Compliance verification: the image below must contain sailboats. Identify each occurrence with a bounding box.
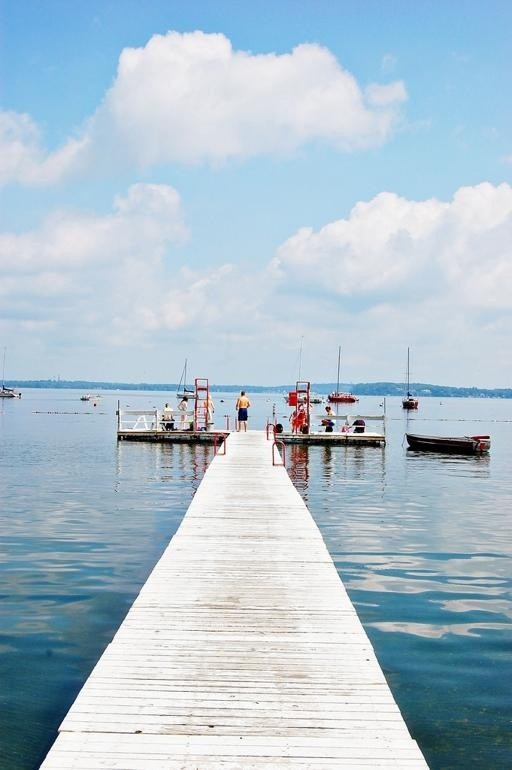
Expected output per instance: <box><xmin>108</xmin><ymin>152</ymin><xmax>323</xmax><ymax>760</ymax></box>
<box><xmin>402</xmin><ymin>347</ymin><xmax>419</xmax><ymax>408</ymax></box>
<box><xmin>176</xmin><ymin>357</ymin><xmax>198</xmax><ymax>398</ymax></box>
<box><xmin>284</xmin><ymin>335</ymin><xmax>359</xmax><ymax>402</ymax></box>
<box><xmin>0</xmin><ymin>347</ymin><xmax>22</xmax><ymax>399</ymax></box>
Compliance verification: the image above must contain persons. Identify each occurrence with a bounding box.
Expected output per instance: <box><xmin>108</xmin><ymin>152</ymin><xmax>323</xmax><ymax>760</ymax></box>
<box><xmin>289</xmin><ymin>397</ymin><xmax>312</xmax><ymax>434</ymax></box>
<box><xmin>320</xmin><ymin>406</ymin><xmax>336</xmax><ymax>432</ymax></box>
<box><xmin>236</xmin><ymin>391</ymin><xmax>251</xmax><ymax>432</ymax></box>
<box><xmin>202</xmin><ymin>395</ymin><xmax>215</xmax><ymax>432</ymax></box>
<box><xmin>161</xmin><ymin>403</ymin><xmax>175</xmax><ymax>431</ymax></box>
<box><xmin>341</xmin><ymin>423</ymin><xmax>351</xmax><ymax>433</ymax></box>
<box><xmin>350</xmin><ymin>415</ymin><xmax>365</xmax><ymax>433</ymax></box>
<box><xmin>177</xmin><ymin>396</ymin><xmax>188</xmax><ymax>430</ymax></box>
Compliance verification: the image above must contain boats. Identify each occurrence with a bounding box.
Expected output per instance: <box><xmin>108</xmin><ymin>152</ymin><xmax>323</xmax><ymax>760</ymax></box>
<box><xmin>405</xmin><ymin>432</ymin><xmax>491</xmax><ymax>454</ymax></box>
<box><xmin>80</xmin><ymin>393</ymin><xmax>105</xmax><ymax>401</ymax></box>
